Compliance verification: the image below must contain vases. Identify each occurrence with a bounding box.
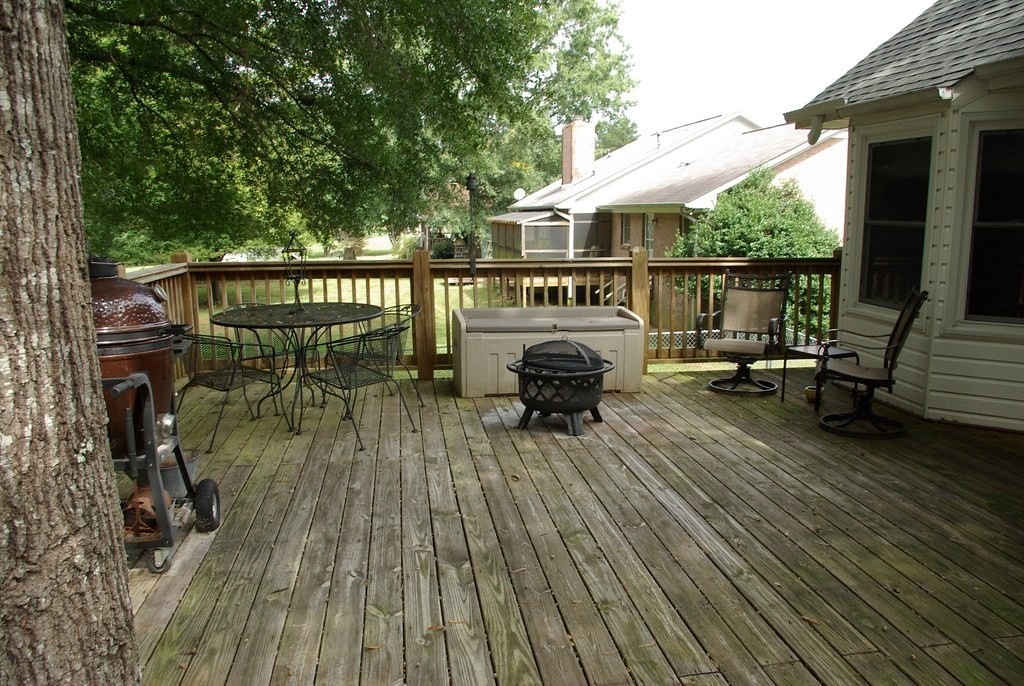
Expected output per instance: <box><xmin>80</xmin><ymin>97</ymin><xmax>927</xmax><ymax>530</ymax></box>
<box><xmin>135</xmin><ymin>450</ymin><xmax>200</xmax><ymax>496</ymax></box>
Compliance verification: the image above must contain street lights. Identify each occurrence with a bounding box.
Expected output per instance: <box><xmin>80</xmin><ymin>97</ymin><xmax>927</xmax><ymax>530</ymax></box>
<box><xmin>463</xmin><ymin>173</ymin><xmax>478</xmax><ymax>257</ymax></box>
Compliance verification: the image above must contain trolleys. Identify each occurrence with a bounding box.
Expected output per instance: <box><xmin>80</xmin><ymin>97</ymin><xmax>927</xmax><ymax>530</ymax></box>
<box><xmin>93</xmin><ymin>371</ymin><xmax>221</xmax><ymax>576</ymax></box>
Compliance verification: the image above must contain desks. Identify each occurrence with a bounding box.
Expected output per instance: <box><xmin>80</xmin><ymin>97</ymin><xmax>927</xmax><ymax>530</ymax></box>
<box><xmin>210</xmin><ymin>302</ymin><xmax>384</xmax><ymax>437</ymax></box>
<box><xmin>782</xmin><ymin>344</ymin><xmax>859</xmax><ymax>408</ymax></box>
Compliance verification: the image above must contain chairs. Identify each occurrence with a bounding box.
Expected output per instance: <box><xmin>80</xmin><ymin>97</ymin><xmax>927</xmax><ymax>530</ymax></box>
<box><xmin>176</xmin><ymin>335</ymin><xmax>291</xmax><ymax>453</ymax></box>
<box><xmin>814</xmin><ymin>283</ymin><xmax>928</xmax><ymax>440</ymax></box>
<box><xmin>223</xmin><ymin>302</ymin><xmax>426</xmax><ymax>451</ymax></box>
<box><xmin>694</xmin><ymin>269</ymin><xmax>792</xmax><ymax>396</ymax></box>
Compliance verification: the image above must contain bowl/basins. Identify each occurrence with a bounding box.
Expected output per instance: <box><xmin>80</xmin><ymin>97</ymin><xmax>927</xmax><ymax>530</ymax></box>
<box><xmin>141</xmin><ymin>448</ymin><xmax>200</xmax><ymax>499</ymax></box>
<box><xmin>806</xmin><ymin>386</ymin><xmax>825</xmax><ymax>403</ymax></box>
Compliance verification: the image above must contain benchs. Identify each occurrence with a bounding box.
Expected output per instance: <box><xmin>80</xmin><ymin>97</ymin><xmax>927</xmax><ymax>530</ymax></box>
<box><xmin>450</xmin><ymin>305</ymin><xmax>645</xmax><ymax>399</ymax></box>
